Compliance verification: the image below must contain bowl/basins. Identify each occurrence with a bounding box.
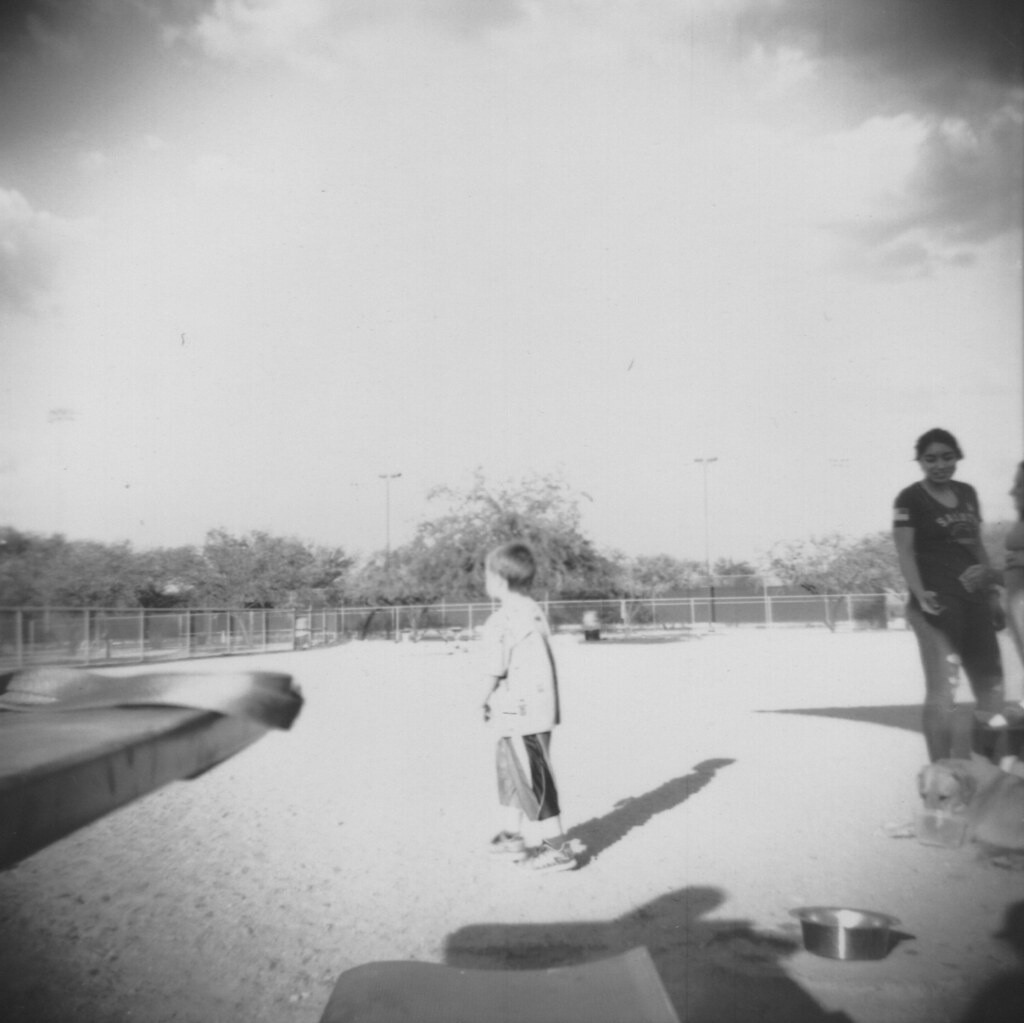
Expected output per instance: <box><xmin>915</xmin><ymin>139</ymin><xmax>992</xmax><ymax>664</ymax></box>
<box><xmin>789</xmin><ymin>907</ymin><xmax>903</xmax><ymax>960</ymax></box>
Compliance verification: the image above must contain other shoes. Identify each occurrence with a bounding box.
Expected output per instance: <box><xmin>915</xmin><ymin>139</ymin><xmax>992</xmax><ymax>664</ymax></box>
<box><xmin>525</xmin><ymin>838</ymin><xmax>578</xmax><ymax>874</ymax></box>
<box><xmin>489</xmin><ymin>831</ymin><xmax>527</xmax><ymax>861</ymax></box>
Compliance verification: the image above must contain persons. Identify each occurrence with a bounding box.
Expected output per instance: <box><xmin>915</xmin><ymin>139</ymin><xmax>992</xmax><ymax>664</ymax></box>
<box><xmin>475</xmin><ymin>544</ymin><xmax>578</xmax><ymax>876</ymax></box>
<box><xmin>1005</xmin><ymin>458</ymin><xmax>1024</xmax><ymax>658</ymax></box>
<box><xmin>894</xmin><ymin>429</ymin><xmax>1024</xmax><ymax>779</ymax></box>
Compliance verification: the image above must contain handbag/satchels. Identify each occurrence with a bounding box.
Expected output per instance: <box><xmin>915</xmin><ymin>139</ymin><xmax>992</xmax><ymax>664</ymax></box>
<box><xmin>944</xmin><ymin>587</ymin><xmax>1007</xmax><ymax>643</ymax></box>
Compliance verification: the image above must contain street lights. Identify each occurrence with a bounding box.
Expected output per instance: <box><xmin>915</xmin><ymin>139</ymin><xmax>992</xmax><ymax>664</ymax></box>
<box><xmin>377</xmin><ymin>469</ymin><xmax>402</xmax><ymax>563</ymax></box>
<box><xmin>692</xmin><ymin>453</ymin><xmax>720</xmax><ymax>582</ymax></box>
